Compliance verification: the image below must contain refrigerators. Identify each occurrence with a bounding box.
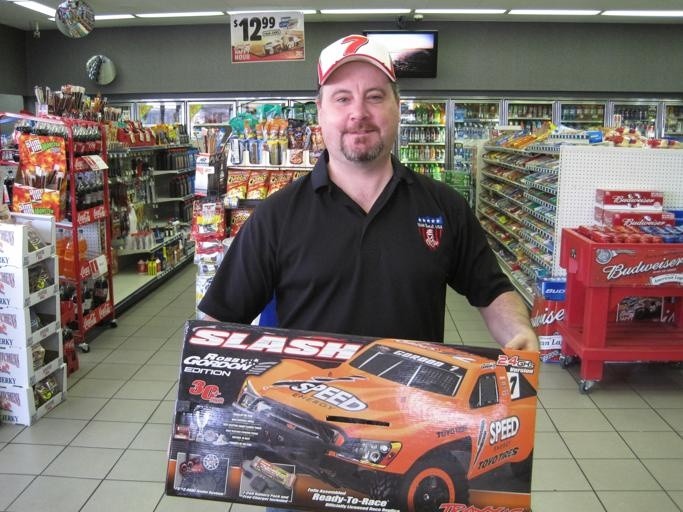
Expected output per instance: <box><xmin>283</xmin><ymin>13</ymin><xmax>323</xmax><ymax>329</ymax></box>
<box><xmin>113</xmin><ymin>98</ymin><xmax>683</xmax><ymax>183</ymax></box>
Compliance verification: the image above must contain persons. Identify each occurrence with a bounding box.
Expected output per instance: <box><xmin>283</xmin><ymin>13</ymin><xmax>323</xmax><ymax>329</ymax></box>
<box><xmin>197</xmin><ymin>34</ymin><xmax>540</xmax><ymax>353</ymax></box>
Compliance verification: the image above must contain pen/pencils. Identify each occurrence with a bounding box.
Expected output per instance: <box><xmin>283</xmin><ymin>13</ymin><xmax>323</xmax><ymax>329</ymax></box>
<box><xmin>35</xmin><ymin>85</ymin><xmax>82</xmax><ymax>116</ymax></box>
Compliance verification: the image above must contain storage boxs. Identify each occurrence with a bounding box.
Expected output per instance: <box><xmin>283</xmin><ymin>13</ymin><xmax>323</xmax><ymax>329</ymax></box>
<box><xmin>166</xmin><ymin>320</ymin><xmax>539</xmax><ymax>512</ymax></box>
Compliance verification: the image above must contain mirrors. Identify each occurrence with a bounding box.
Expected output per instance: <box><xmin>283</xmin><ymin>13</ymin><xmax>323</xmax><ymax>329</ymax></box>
<box><xmin>86</xmin><ymin>55</ymin><xmax>117</xmax><ymax>86</ymax></box>
<box><xmin>55</xmin><ymin>0</ymin><xmax>95</xmax><ymax>39</ymax></box>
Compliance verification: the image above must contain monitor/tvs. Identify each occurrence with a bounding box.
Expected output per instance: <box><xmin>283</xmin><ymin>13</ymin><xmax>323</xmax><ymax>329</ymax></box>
<box><xmin>362</xmin><ymin>28</ymin><xmax>439</xmax><ymax>79</ymax></box>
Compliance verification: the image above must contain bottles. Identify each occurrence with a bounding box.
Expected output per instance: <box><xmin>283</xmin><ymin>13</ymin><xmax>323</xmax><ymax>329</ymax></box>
<box><xmin>136</xmin><ymin>124</ymin><xmax>197</xmax><ymax>276</ymax></box>
<box><xmin>2</xmin><ymin>111</ymin><xmax>112</xmax><ymax>376</ymax></box>
<box><xmin>399</xmin><ymin>105</ymin><xmax>651</xmax><ymax>179</ymax></box>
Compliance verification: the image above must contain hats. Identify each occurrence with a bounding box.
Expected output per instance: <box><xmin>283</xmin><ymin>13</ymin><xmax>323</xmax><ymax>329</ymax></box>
<box><xmin>317</xmin><ymin>34</ymin><xmax>397</xmax><ymax>86</ymax></box>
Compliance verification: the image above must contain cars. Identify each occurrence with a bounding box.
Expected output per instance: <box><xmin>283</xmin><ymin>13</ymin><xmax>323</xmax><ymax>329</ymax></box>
<box><xmin>231</xmin><ymin>340</ymin><xmax>536</xmax><ymax>512</ymax></box>
